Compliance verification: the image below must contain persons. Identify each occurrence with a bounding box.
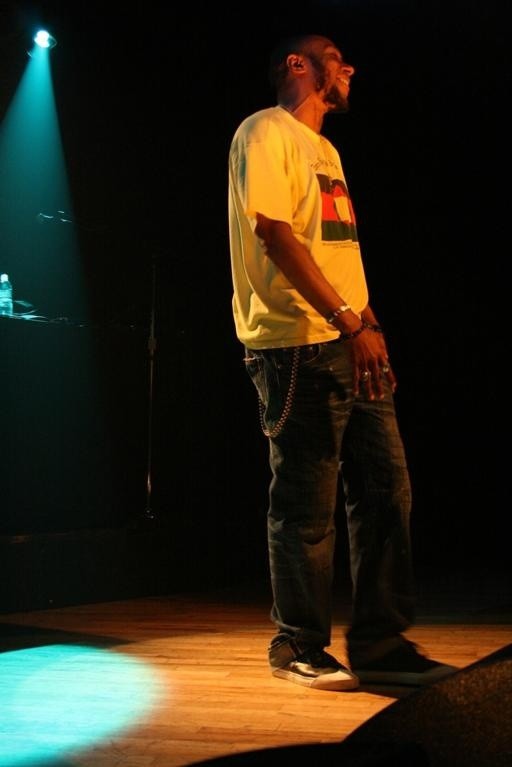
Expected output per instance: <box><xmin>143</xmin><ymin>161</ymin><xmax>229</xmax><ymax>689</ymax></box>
<box><xmin>226</xmin><ymin>33</ymin><xmax>461</xmax><ymax>691</ymax></box>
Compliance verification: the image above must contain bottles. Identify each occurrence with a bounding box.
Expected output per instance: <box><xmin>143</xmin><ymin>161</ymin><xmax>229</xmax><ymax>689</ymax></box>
<box><xmin>0</xmin><ymin>273</ymin><xmax>14</xmax><ymax>317</ymax></box>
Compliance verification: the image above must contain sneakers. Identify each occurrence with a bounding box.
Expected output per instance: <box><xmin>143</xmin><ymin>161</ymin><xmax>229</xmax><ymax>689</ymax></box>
<box><xmin>270</xmin><ymin>647</ymin><xmax>361</xmax><ymax>692</ymax></box>
<box><xmin>348</xmin><ymin>643</ymin><xmax>459</xmax><ymax>688</ymax></box>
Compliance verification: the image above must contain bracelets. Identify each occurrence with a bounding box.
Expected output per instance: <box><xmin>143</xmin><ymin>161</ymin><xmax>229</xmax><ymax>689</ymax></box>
<box><xmin>337</xmin><ymin>321</ymin><xmax>368</xmax><ymax>341</ymax></box>
<box><xmin>326</xmin><ymin>304</ymin><xmax>350</xmax><ymax>323</ymax></box>
<box><xmin>368</xmin><ymin>323</ymin><xmax>382</xmax><ymax>333</ymax></box>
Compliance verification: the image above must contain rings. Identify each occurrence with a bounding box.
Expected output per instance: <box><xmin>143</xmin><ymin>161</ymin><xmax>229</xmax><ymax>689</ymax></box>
<box><xmin>360</xmin><ymin>370</ymin><xmax>373</xmax><ymax>383</ymax></box>
<box><xmin>380</xmin><ymin>361</ymin><xmax>391</xmax><ymax>377</ymax></box>
